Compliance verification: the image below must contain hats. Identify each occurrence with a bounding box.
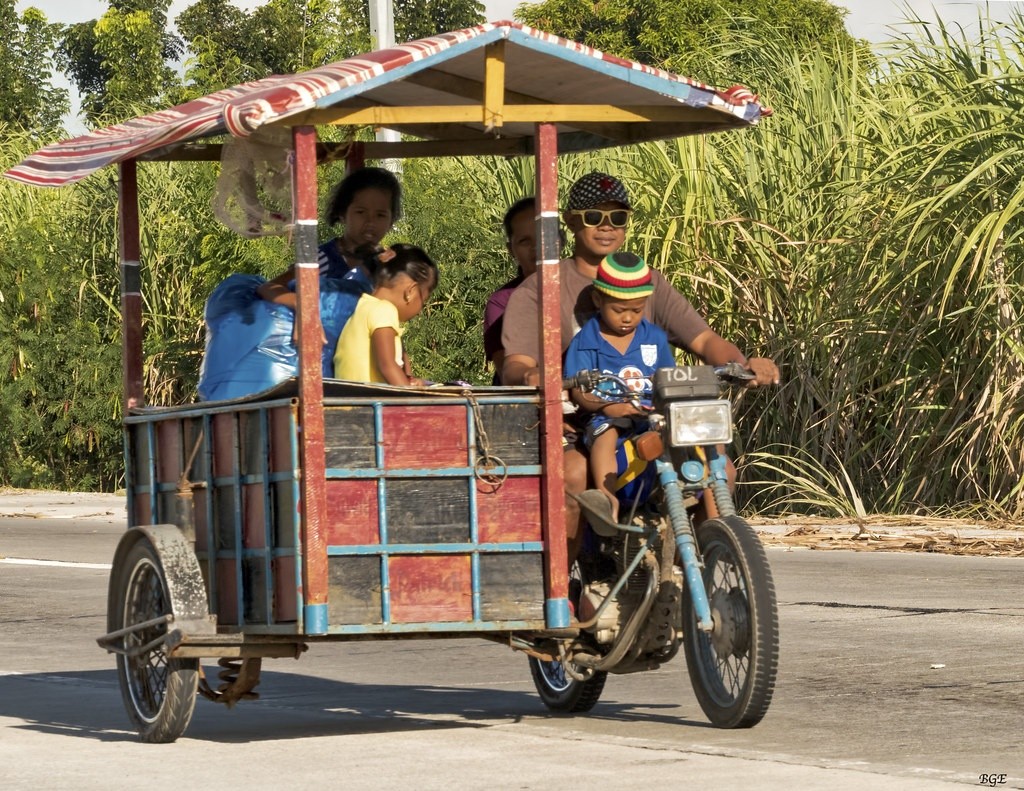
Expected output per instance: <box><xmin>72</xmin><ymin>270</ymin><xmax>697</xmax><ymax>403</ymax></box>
<box><xmin>592</xmin><ymin>252</ymin><xmax>654</xmax><ymax>300</ymax></box>
<box><xmin>567</xmin><ymin>172</ymin><xmax>632</xmax><ymax>210</ymax></box>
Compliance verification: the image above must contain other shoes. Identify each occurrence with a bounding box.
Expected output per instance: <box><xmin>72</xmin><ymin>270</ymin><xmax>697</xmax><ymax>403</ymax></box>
<box><xmin>580</xmin><ymin>489</ymin><xmax>621</xmax><ymax>536</ymax></box>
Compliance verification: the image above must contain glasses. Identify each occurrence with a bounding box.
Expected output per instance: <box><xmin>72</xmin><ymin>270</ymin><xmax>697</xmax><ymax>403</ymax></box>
<box><xmin>570</xmin><ymin>209</ymin><xmax>631</xmax><ymax>227</ymax></box>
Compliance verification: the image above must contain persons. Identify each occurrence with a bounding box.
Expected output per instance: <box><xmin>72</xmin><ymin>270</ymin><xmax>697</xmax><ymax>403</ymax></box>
<box><xmin>482</xmin><ymin>198</ymin><xmax>563</xmax><ymax>387</ymax></box>
<box><xmin>257</xmin><ymin>167</ymin><xmax>402</xmax><ymax>350</ymax></box>
<box><xmin>564</xmin><ymin>251</ymin><xmax>675</xmax><ymax>537</ymax></box>
<box><xmin>332</xmin><ymin>243</ymin><xmax>439</xmax><ymax>389</ymax></box>
<box><xmin>500</xmin><ymin>168</ymin><xmax>780</xmax><ymax>627</ymax></box>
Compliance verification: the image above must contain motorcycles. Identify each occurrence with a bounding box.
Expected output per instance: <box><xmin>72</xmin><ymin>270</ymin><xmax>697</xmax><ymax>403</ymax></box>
<box><xmin>4</xmin><ymin>18</ymin><xmax>781</xmax><ymax>749</ymax></box>
